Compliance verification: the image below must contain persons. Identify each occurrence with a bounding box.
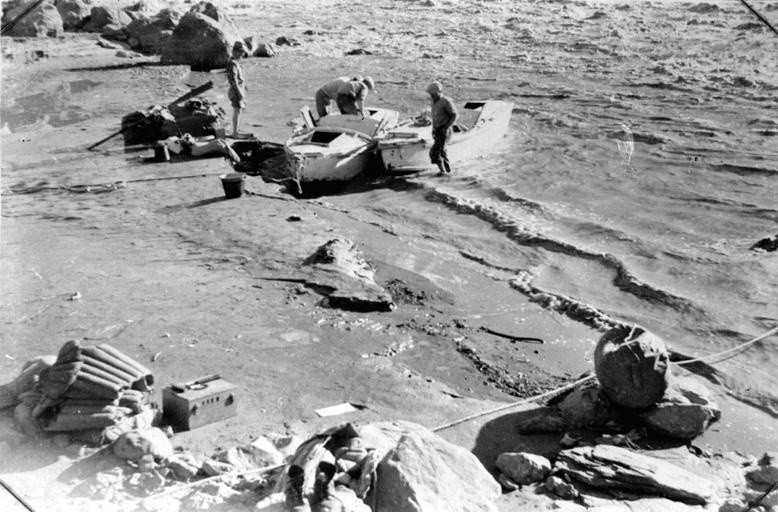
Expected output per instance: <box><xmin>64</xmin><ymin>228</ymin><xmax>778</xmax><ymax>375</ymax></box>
<box><xmin>336</xmin><ymin>75</ymin><xmax>375</xmax><ymax>117</ymax></box>
<box><xmin>226</xmin><ymin>41</ymin><xmax>249</xmax><ymax>140</ymax></box>
<box><xmin>316</xmin><ymin>73</ymin><xmax>361</xmax><ymax>117</ymax></box>
<box><xmin>425</xmin><ymin>81</ymin><xmax>458</xmax><ymax>176</ymax></box>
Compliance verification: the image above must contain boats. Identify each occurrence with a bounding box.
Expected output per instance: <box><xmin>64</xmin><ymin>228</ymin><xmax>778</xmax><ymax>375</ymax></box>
<box><xmin>122</xmin><ymin>104</ymin><xmax>217</xmax><ymax>146</ymax></box>
<box><xmin>284</xmin><ymin>105</ymin><xmax>399</xmax><ymax>182</ymax></box>
<box><xmin>377</xmin><ymin>100</ymin><xmax>512</xmax><ymax>170</ymax></box>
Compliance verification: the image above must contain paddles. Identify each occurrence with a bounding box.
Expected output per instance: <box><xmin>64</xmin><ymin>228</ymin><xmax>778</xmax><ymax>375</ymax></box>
<box><xmin>87</xmin><ymin>80</ymin><xmax>213</xmax><ymax>150</ymax></box>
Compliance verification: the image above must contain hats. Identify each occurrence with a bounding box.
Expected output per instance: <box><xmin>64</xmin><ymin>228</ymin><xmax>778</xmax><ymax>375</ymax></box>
<box><xmin>426</xmin><ymin>81</ymin><xmax>442</xmax><ymax>94</ymax></box>
<box><xmin>353</xmin><ymin>75</ymin><xmax>374</xmax><ymax>90</ymax></box>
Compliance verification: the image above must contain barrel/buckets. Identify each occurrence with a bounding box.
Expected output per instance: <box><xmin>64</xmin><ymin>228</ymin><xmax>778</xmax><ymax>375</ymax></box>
<box><xmin>155</xmin><ymin>143</ymin><xmax>170</xmax><ymax>162</ymax></box>
<box><xmin>220</xmin><ymin>173</ymin><xmax>246</xmax><ymax>198</ymax></box>
